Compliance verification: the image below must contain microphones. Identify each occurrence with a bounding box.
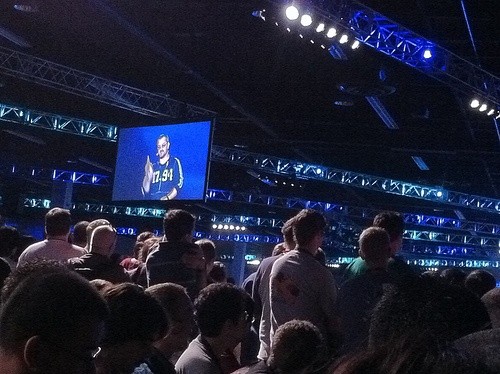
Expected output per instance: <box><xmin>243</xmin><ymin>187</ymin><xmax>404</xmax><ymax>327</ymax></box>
<box><xmin>156</xmin><ymin>153</ymin><xmax>159</xmax><ymax>156</ymax></box>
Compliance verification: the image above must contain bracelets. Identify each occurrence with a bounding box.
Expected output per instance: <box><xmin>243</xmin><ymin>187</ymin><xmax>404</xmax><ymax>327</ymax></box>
<box><xmin>166</xmin><ymin>195</ymin><xmax>169</xmax><ymax>200</ymax></box>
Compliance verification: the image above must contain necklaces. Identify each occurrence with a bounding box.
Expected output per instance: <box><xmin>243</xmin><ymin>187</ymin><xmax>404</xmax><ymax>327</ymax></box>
<box><xmin>48</xmin><ymin>238</ymin><xmax>67</xmax><ymax>242</ymax></box>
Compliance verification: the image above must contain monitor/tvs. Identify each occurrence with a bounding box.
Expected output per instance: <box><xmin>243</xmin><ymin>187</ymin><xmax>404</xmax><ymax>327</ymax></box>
<box><xmin>112</xmin><ymin>117</ymin><xmax>215</xmax><ymax>205</ymax></box>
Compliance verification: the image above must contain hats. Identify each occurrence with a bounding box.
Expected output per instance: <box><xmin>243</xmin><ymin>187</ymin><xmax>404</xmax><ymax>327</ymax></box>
<box><xmin>281</xmin><ymin>216</ymin><xmax>296</xmax><ymax>234</ymax></box>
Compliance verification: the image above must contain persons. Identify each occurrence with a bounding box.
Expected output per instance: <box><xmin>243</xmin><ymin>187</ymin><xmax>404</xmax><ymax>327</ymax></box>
<box><xmin>140</xmin><ymin>132</ymin><xmax>185</xmax><ymax>200</ymax></box>
<box><xmin>0</xmin><ymin>207</ymin><xmax>500</xmax><ymax>374</ymax></box>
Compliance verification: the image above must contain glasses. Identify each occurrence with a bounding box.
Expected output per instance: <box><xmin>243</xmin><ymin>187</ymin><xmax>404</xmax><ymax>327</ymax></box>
<box><xmin>156</xmin><ymin>142</ymin><xmax>167</xmax><ymax>150</ymax></box>
<box><xmin>27</xmin><ymin>331</ymin><xmax>102</xmax><ymax>364</ymax></box>
<box><xmin>224</xmin><ymin>314</ymin><xmax>255</xmax><ymax>326</ymax></box>
